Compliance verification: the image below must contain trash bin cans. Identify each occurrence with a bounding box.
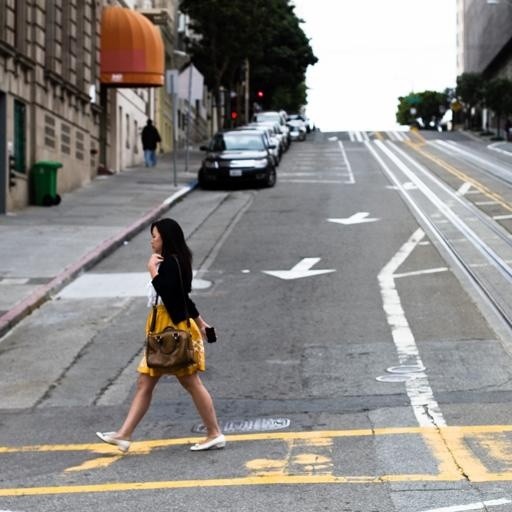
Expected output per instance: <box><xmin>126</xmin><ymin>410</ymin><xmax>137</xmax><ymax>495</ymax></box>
<box><xmin>30</xmin><ymin>161</ymin><xmax>61</xmax><ymax>206</ymax></box>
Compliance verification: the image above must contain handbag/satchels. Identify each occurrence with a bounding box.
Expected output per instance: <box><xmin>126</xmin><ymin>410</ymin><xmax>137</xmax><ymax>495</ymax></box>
<box><xmin>146</xmin><ymin>326</ymin><xmax>196</xmax><ymax>368</ymax></box>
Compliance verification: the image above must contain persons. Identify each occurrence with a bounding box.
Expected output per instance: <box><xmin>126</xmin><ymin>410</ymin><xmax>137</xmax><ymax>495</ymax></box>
<box><xmin>95</xmin><ymin>217</ymin><xmax>226</xmax><ymax>453</ymax></box>
<box><xmin>139</xmin><ymin>117</ymin><xmax>165</xmax><ymax>168</ymax></box>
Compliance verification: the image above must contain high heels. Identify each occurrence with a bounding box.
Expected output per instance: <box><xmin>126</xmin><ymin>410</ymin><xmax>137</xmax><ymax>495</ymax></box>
<box><xmin>190</xmin><ymin>433</ymin><xmax>226</xmax><ymax>451</ymax></box>
<box><xmin>96</xmin><ymin>431</ymin><xmax>132</xmax><ymax>453</ymax></box>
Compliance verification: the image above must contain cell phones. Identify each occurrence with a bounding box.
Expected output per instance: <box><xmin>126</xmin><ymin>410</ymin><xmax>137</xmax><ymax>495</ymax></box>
<box><xmin>205</xmin><ymin>327</ymin><xmax>216</xmax><ymax>343</ymax></box>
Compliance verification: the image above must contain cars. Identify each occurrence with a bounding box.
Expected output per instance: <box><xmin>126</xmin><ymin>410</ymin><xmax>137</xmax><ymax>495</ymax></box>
<box><xmin>232</xmin><ymin>106</ymin><xmax>310</xmax><ymax>164</ymax></box>
<box><xmin>196</xmin><ymin>126</ymin><xmax>279</xmax><ymax>190</ymax></box>
<box><xmin>409</xmin><ymin>112</ymin><xmax>449</xmax><ymax>133</ymax></box>
